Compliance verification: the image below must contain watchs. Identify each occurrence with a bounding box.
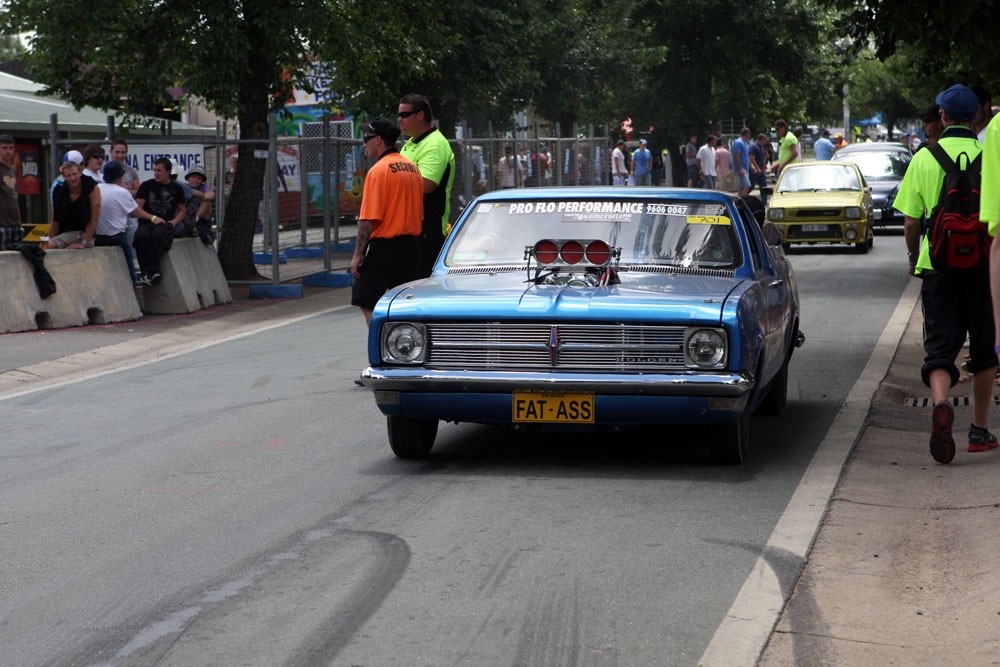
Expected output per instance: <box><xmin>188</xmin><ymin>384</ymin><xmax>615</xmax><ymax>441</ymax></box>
<box><xmin>907</xmin><ymin>250</ymin><xmax>920</xmax><ymax>265</ymax></box>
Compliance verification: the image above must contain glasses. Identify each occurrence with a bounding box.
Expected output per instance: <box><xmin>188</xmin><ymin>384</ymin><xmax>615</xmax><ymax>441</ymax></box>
<box><xmin>363</xmin><ymin>134</ymin><xmax>377</xmax><ymax>143</ymax></box>
<box><xmin>86</xmin><ymin>153</ymin><xmax>105</xmax><ymax>160</ymax></box>
<box><xmin>923</xmin><ymin>115</ymin><xmax>939</xmax><ymax>123</ymax></box>
<box><xmin>398</xmin><ymin>109</ymin><xmax>422</xmax><ymax>118</ymax></box>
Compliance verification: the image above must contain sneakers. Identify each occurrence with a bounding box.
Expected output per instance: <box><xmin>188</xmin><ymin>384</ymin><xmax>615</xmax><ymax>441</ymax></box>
<box><xmin>929</xmin><ymin>400</ymin><xmax>956</xmax><ymax>464</ymax></box>
<box><xmin>968</xmin><ymin>423</ymin><xmax>998</xmax><ymax>452</ymax></box>
<box><xmin>151</xmin><ymin>270</ymin><xmax>162</xmax><ymax>286</ymax></box>
<box><xmin>140</xmin><ymin>271</ymin><xmax>152</xmax><ymax>288</ymax></box>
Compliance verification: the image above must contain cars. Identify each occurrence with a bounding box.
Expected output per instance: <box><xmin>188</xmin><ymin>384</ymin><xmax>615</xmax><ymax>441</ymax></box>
<box><xmin>363</xmin><ymin>181</ymin><xmax>801</xmax><ymax>467</ymax></box>
<box><xmin>831</xmin><ymin>141</ymin><xmax>915</xmax><ymax>233</ymax></box>
<box><xmin>759</xmin><ymin>160</ymin><xmax>876</xmax><ymax>254</ymax></box>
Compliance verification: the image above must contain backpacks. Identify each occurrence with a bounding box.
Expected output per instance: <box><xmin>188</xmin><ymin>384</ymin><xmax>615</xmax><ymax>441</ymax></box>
<box><xmin>923</xmin><ymin>142</ymin><xmax>993</xmax><ymax>273</ymax></box>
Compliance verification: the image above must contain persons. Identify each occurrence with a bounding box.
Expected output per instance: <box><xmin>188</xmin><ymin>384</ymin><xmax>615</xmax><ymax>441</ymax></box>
<box><xmin>978</xmin><ymin>110</ymin><xmax>1000</xmax><ymax>365</ymax></box>
<box><xmin>970</xmin><ymin>84</ymin><xmax>995</xmax><ymax>144</ymax></box>
<box><xmin>396</xmin><ymin>92</ymin><xmax>457</xmax><ymax>270</ymax></box>
<box><xmin>917</xmin><ymin>104</ymin><xmax>946</xmax><ymax>153</ymax></box>
<box><xmin>0</xmin><ymin>132</ymin><xmax>216</xmax><ymax>287</ymax></box>
<box><xmin>349</xmin><ymin>116</ymin><xmax>426</xmax><ymax>388</ymax></box>
<box><xmin>892</xmin><ymin>84</ymin><xmax>1000</xmax><ymax>464</ymax></box>
<box><xmin>498</xmin><ymin>118</ymin><xmax>922</xmax><ymax>197</ymax></box>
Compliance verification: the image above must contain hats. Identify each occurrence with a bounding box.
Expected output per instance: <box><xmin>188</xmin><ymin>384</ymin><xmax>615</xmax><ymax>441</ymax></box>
<box><xmin>103</xmin><ymin>160</ymin><xmax>125</xmax><ymax>182</ymax></box>
<box><xmin>935</xmin><ymin>83</ymin><xmax>980</xmax><ymax>121</ymax></box>
<box><xmin>359</xmin><ymin>117</ymin><xmax>401</xmax><ymax>141</ymax></box>
<box><xmin>59</xmin><ymin>150</ymin><xmax>84</xmax><ymax>173</ymax></box>
<box><xmin>169</xmin><ymin>158</ymin><xmax>184</xmax><ymax>175</ymax></box>
<box><xmin>185</xmin><ymin>165</ymin><xmax>207</xmax><ymax>182</ymax></box>
